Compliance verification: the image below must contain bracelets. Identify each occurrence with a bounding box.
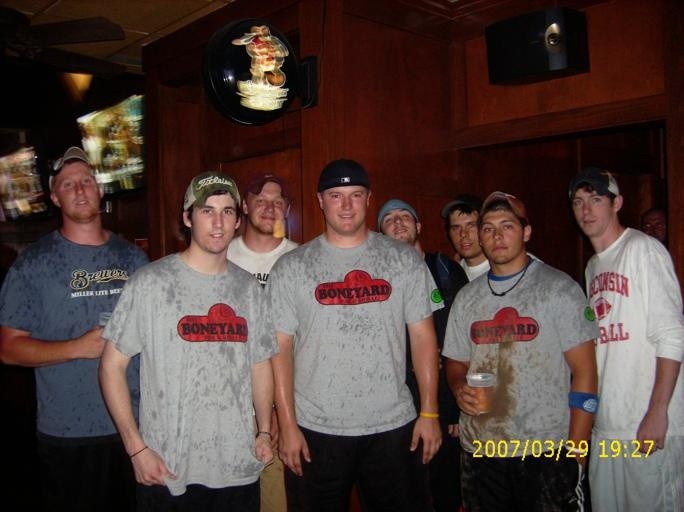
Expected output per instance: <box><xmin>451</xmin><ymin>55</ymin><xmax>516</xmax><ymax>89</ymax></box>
<box><xmin>564</xmin><ymin>389</ymin><xmax>600</xmax><ymax>414</ymax></box>
<box><xmin>252</xmin><ymin>431</ymin><xmax>276</xmax><ymax>439</ymax></box>
<box><xmin>417</xmin><ymin>412</ymin><xmax>445</xmax><ymax>420</ymax></box>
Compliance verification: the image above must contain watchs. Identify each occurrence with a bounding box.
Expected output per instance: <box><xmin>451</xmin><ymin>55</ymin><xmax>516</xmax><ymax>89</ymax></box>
<box><xmin>130</xmin><ymin>444</ymin><xmax>153</xmax><ymax>461</ymax></box>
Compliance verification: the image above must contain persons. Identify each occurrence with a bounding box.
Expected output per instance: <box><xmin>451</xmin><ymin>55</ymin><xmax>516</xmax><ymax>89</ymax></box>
<box><xmin>261</xmin><ymin>159</ymin><xmax>447</xmax><ymax>512</ymax></box>
<box><xmin>377</xmin><ymin>196</ymin><xmax>469</xmax><ymax>512</ymax></box>
<box><xmin>0</xmin><ymin>146</ymin><xmax>155</xmax><ymax>512</ymax></box>
<box><xmin>439</xmin><ymin>192</ymin><xmax>603</xmax><ymax>511</ymax></box>
<box><xmin>224</xmin><ymin>171</ymin><xmax>301</xmax><ymax>512</ymax></box>
<box><xmin>564</xmin><ymin>156</ymin><xmax>683</xmax><ymax>512</ymax></box>
<box><xmin>641</xmin><ymin>204</ymin><xmax>669</xmax><ymax>250</ymax></box>
<box><xmin>98</xmin><ymin>171</ymin><xmax>282</xmax><ymax>512</ymax></box>
<box><xmin>439</xmin><ymin>192</ymin><xmax>542</xmax><ymax>294</ymax></box>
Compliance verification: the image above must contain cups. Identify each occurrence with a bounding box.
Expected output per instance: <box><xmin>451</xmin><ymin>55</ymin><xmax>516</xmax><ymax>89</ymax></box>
<box><xmin>464</xmin><ymin>371</ymin><xmax>498</xmax><ymax>415</ymax></box>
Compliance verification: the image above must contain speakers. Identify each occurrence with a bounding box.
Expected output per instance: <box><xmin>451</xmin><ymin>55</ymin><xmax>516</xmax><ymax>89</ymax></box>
<box><xmin>484</xmin><ymin>8</ymin><xmax>589</xmax><ymax>85</ymax></box>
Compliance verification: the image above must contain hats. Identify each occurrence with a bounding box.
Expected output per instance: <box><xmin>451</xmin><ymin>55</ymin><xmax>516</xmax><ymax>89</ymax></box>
<box><xmin>377</xmin><ymin>199</ymin><xmax>419</xmax><ymax>234</ymax></box>
<box><xmin>568</xmin><ymin>167</ymin><xmax>619</xmax><ymax>200</ymax></box>
<box><xmin>48</xmin><ymin>146</ymin><xmax>96</xmax><ymax>192</ymax></box>
<box><xmin>479</xmin><ymin>191</ymin><xmax>529</xmax><ymax>224</ymax></box>
<box><xmin>245</xmin><ymin>171</ymin><xmax>288</xmax><ymax>198</ymax></box>
<box><xmin>183</xmin><ymin>170</ymin><xmax>240</xmax><ymax>212</ymax></box>
<box><xmin>318</xmin><ymin>159</ymin><xmax>369</xmax><ymax>193</ymax></box>
<box><xmin>440</xmin><ymin>193</ymin><xmax>480</xmax><ymax>219</ymax></box>
